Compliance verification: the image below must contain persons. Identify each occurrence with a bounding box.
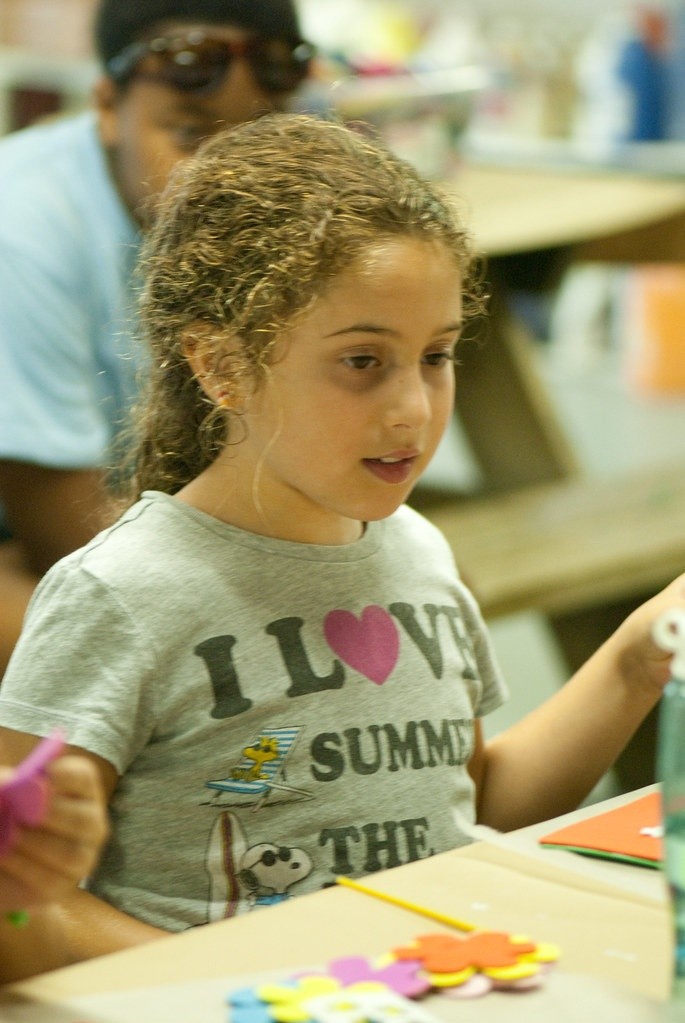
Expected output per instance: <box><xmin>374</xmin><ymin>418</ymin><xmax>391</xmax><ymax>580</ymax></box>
<box><xmin>0</xmin><ymin>115</ymin><xmax>685</xmax><ymax>983</ymax></box>
<box><xmin>0</xmin><ymin>0</ymin><xmax>314</xmax><ymax>682</ymax></box>
<box><xmin>0</xmin><ymin>756</ymin><xmax>105</xmax><ymax>916</ymax></box>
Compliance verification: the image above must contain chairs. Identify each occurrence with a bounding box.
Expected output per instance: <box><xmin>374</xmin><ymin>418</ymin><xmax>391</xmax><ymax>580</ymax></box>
<box><xmin>439</xmin><ymin>462</ymin><xmax>685</xmax><ymax>618</ymax></box>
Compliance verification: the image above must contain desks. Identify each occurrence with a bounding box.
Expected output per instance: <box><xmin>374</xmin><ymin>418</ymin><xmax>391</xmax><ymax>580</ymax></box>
<box><xmin>427</xmin><ymin>173</ymin><xmax>685</xmax><ymax>795</ymax></box>
<box><xmin>0</xmin><ymin>780</ymin><xmax>672</xmax><ymax>1023</ymax></box>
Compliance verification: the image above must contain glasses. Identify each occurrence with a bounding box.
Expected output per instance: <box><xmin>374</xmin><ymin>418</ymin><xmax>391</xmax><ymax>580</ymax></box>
<box><xmin>109</xmin><ymin>27</ymin><xmax>318</xmax><ymax>96</ymax></box>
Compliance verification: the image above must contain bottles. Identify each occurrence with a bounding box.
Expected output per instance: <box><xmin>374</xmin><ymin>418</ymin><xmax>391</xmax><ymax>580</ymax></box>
<box><xmin>654</xmin><ymin>612</ymin><xmax>685</xmax><ymax>997</ymax></box>
